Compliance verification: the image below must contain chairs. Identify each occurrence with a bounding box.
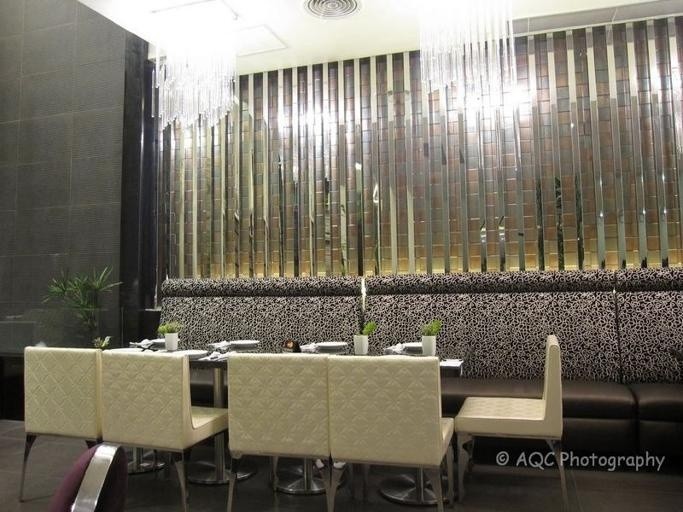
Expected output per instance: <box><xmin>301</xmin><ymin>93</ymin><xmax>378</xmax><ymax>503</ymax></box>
<box><xmin>18</xmin><ymin>346</ymin><xmax>457</xmax><ymax>512</ymax></box>
<box><xmin>453</xmin><ymin>331</ymin><xmax>577</xmax><ymax>511</ymax></box>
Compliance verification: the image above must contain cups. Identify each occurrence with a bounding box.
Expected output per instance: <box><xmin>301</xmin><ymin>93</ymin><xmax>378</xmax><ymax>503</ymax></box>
<box><xmin>422</xmin><ymin>335</ymin><xmax>436</xmax><ymax>356</ymax></box>
<box><xmin>354</xmin><ymin>334</ymin><xmax>368</xmax><ymax>355</ymax></box>
<box><xmin>164</xmin><ymin>333</ymin><xmax>178</xmax><ymax>351</ymax></box>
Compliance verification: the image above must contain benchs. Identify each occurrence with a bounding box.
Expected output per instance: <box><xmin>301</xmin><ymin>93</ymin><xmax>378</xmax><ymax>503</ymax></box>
<box><xmin>154</xmin><ymin>265</ymin><xmax>683</xmax><ymax>464</ymax></box>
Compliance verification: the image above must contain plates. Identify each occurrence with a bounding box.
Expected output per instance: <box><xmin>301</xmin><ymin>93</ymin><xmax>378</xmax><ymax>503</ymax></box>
<box><xmin>403</xmin><ymin>342</ymin><xmax>422</xmax><ymax>351</ymax></box>
<box><xmin>314</xmin><ymin>342</ymin><xmax>347</xmax><ymax>350</ymax></box>
<box><xmin>110</xmin><ymin>339</ymin><xmax>260</xmax><ymax>359</ymax></box>
